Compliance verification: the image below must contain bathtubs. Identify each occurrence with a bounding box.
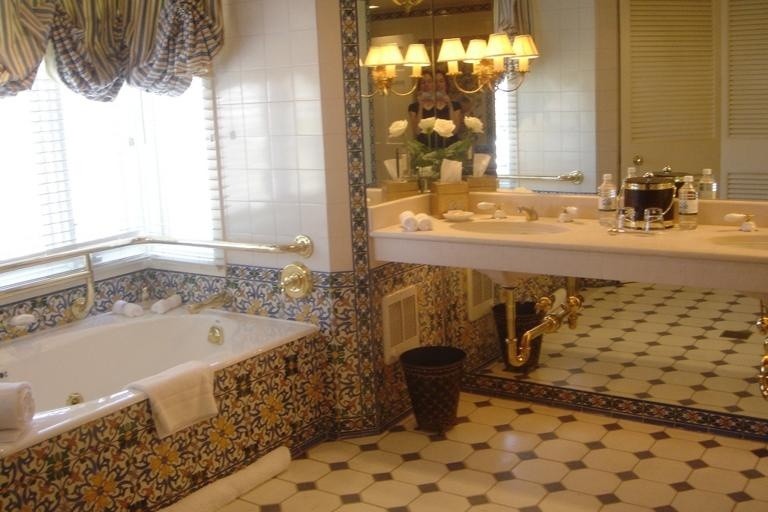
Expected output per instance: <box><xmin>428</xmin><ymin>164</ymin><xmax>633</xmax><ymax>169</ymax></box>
<box><xmin>0</xmin><ymin>299</ymin><xmax>330</xmax><ymax>512</ymax></box>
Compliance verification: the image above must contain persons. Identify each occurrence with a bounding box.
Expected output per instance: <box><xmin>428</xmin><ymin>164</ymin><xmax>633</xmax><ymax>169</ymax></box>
<box><xmin>408</xmin><ymin>70</ymin><xmax>462</xmax><ymax>149</ymax></box>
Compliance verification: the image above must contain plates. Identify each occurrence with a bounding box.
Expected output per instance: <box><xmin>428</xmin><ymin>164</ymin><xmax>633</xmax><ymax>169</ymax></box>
<box><xmin>442</xmin><ymin>211</ymin><xmax>475</xmax><ymax>222</ymax></box>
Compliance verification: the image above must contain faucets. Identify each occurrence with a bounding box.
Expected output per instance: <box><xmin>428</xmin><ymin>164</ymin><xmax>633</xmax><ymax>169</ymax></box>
<box><xmin>185</xmin><ymin>291</ymin><xmax>231</xmax><ymax>315</ymax></box>
<box><xmin>515</xmin><ymin>205</ymin><xmax>539</xmax><ymax>221</ymax></box>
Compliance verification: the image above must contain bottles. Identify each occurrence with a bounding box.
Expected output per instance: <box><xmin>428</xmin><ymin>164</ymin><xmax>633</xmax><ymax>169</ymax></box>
<box><xmin>678</xmin><ymin>175</ymin><xmax>700</xmax><ymax>231</ymax></box>
<box><xmin>627</xmin><ymin>166</ymin><xmax>637</xmax><ymax>179</ymax></box>
<box><xmin>597</xmin><ymin>172</ymin><xmax>617</xmax><ymax>225</ymax></box>
<box><xmin>699</xmin><ymin>168</ymin><xmax>717</xmax><ymax>200</ymax></box>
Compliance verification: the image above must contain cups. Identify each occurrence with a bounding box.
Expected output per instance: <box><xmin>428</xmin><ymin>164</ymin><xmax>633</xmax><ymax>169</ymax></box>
<box><xmin>643</xmin><ymin>207</ymin><xmax>666</xmax><ymax>233</ymax></box>
<box><xmin>616</xmin><ymin>206</ymin><xmax>637</xmax><ymax>233</ymax></box>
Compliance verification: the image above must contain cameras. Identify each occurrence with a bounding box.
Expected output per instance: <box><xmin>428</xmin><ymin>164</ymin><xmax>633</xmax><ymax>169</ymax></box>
<box><xmin>436</xmin><ymin>90</ymin><xmax>445</xmax><ymax>102</ymax></box>
<box><xmin>417</xmin><ymin>90</ymin><xmax>429</xmax><ymax>104</ymax></box>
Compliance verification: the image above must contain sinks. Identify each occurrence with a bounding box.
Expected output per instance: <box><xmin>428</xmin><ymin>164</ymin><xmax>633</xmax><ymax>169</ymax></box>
<box><xmin>711</xmin><ymin>232</ymin><xmax>767</xmax><ymax>250</ymax></box>
<box><xmin>450</xmin><ymin>217</ymin><xmax>572</xmax><ymax>237</ymax></box>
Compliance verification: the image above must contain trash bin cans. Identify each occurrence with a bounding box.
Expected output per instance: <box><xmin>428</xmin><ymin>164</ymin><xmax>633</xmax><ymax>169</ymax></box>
<box><xmin>492</xmin><ymin>301</ymin><xmax>545</xmax><ymax>371</ymax></box>
<box><xmin>400</xmin><ymin>346</ymin><xmax>467</xmax><ymax>432</ymax></box>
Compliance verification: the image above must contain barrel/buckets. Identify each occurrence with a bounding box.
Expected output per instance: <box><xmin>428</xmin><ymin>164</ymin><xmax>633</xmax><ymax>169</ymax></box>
<box><xmin>619</xmin><ymin>176</ymin><xmax>678</xmax><ymax>232</ymax></box>
<box><xmin>653</xmin><ymin>170</ymin><xmax>697</xmax><ymax>199</ymax></box>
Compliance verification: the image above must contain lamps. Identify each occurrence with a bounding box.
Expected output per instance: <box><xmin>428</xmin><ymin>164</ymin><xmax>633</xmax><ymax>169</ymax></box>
<box><xmin>437</xmin><ymin>32</ymin><xmax>539</xmax><ymax>94</ymax></box>
<box><xmin>361</xmin><ymin>44</ymin><xmax>431</xmax><ymax>98</ymax></box>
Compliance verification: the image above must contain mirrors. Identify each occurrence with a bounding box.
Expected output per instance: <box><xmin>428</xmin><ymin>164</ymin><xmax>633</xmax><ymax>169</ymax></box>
<box><xmin>357</xmin><ymin>0</ymin><xmax>768</xmax><ymax>206</ymax></box>
<box><xmin>443</xmin><ymin>266</ymin><xmax>768</xmax><ymax>419</ymax></box>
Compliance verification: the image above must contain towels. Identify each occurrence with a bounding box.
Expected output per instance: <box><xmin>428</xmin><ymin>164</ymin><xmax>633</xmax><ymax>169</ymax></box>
<box><xmin>399</xmin><ymin>211</ymin><xmax>418</xmax><ymax>232</ymax></box>
<box><xmin>125</xmin><ymin>358</ymin><xmax>219</xmax><ymax>440</ymax></box>
<box><xmin>416</xmin><ymin>213</ymin><xmax>433</xmax><ymax>231</ymax></box>
<box><xmin>0</xmin><ymin>382</ymin><xmax>35</xmax><ymax>442</ymax></box>
<box><xmin>112</xmin><ymin>294</ymin><xmax>182</xmax><ymax>317</ymax></box>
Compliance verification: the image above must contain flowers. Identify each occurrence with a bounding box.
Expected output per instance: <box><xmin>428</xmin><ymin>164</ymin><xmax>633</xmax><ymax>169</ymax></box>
<box><xmin>388</xmin><ymin>116</ymin><xmax>484</xmax><ymax>171</ymax></box>
